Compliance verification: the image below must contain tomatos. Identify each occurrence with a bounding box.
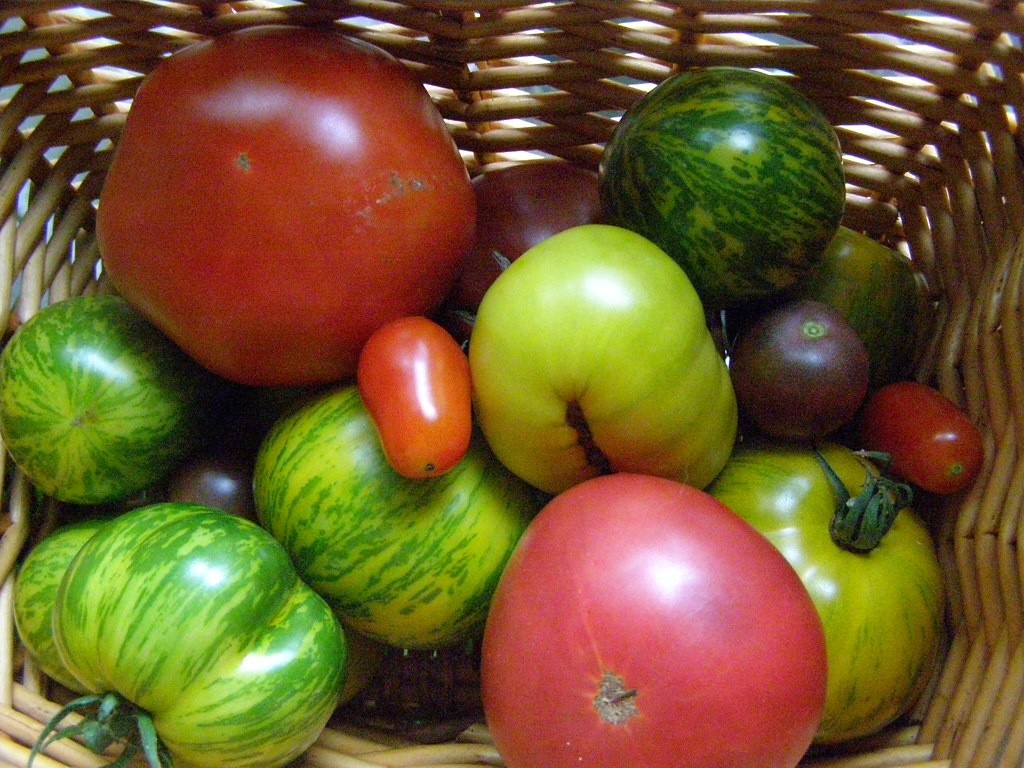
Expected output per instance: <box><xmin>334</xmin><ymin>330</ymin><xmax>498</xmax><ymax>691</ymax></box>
<box><xmin>0</xmin><ymin>21</ymin><xmax>986</xmax><ymax>768</ymax></box>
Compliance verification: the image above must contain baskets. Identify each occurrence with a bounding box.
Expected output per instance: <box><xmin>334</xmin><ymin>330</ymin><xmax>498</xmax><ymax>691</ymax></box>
<box><xmin>0</xmin><ymin>0</ymin><xmax>1024</xmax><ymax>768</ymax></box>
